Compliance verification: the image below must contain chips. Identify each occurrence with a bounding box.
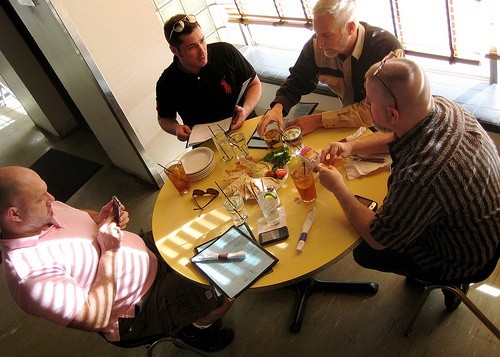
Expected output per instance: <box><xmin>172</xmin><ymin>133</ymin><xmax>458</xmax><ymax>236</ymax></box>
<box><xmin>220</xmin><ymin>156</ymin><xmax>261</xmax><ymax>201</ymax></box>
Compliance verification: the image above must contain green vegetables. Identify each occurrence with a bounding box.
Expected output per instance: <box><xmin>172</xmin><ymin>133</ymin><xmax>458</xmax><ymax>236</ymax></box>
<box><xmin>263</xmin><ymin>146</ymin><xmax>291</xmax><ymax>170</ymax></box>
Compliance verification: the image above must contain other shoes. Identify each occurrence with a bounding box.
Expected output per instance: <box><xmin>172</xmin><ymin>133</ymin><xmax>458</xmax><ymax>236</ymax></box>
<box><xmin>172</xmin><ymin>324</ymin><xmax>235</xmax><ymax>353</ymax></box>
<box><xmin>404</xmin><ymin>276</ymin><xmax>434</xmax><ymax>288</ymax></box>
<box><xmin>442</xmin><ymin>283</ymin><xmax>469</xmax><ymax>312</ymax></box>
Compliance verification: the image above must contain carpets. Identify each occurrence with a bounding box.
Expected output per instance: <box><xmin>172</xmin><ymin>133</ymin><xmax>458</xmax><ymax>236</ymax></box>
<box><xmin>28</xmin><ymin>148</ymin><xmax>104</xmax><ymax>204</ymax></box>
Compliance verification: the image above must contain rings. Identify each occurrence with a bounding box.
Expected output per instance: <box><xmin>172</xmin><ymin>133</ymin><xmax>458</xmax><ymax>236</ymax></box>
<box><xmin>114</xmin><ymin>227</ymin><xmax>121</xmax><ymax>232</ymax></box>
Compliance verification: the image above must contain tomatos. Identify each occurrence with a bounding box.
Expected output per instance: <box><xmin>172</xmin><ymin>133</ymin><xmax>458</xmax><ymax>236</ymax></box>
<box><xmin>275</xmin><ymin>168</ymin><xmax>287</xmax><ymax>177</ymax></box>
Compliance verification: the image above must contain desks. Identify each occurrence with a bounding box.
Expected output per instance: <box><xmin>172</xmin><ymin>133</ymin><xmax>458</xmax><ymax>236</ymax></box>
<box><xmin>151</xmin><ymin>110</ymin><xmax>393</xmax><ymax>334</ymax></box>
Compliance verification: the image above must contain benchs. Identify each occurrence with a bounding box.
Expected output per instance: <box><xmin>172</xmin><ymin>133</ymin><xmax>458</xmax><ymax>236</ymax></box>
<box><xmin>230</xmin><ymin>43</ymin><xmax>500</xmax><ymax>157</ymax></box>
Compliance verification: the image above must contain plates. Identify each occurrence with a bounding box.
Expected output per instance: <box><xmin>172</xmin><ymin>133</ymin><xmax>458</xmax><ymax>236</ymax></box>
<box><xmin>179</xmin><ymin>147</ymin><xmax>216</xmax><ymax>181</ymax></box>
<box><xmin>245</xmin><ymin>173</ymin><xmax>288</xmax><ymax>199</ymax></box>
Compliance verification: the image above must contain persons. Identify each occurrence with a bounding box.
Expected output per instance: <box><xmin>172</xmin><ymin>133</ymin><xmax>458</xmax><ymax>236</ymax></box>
<box><xmin>155</xmin><ymin>14</ymin><xmax>262</xmax><ymax>149</ymax></box>
<box><xmin>0</xmin><ymin>166</ymin><xmax>234</xmax><ymax>353</ymax></box>
<box><xmin>257</xmin><ymin>0</ymin><xmax>405</xmax><ymax>136</ymax></box>
<box><xmin>315</xmin><ymin>51</ymin><xmax>500</xmax><ymax>311</ymax></box>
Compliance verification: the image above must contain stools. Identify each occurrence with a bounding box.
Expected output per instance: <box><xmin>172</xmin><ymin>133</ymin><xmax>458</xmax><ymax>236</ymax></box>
<box><xmin>401</xmin><ymin>244</ymin><xmax>500</xmax><ymax>339</ymax></box>
<box><xmin>125</xmin><ymin>325</ymin><xmax>210</xmax><ymax>357</ymax></box>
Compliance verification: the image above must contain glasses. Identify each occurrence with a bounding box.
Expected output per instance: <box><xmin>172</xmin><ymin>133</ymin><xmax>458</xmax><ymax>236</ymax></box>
<box><xmin>373</xmin><ymin>51</ymin><xmax>398</xmax><ymax>111</ymax></box>
<box><xmin>169</xmin><ymin>13</ymin><xmax>196</xmax><ymax>39</ymax></box>
<box><xmin>192</xmin><ymin>187</ymin><xmax>219</xmax><ymax>211</ymax></box>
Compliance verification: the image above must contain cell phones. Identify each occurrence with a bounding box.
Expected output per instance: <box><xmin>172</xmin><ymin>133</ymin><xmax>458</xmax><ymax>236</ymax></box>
<box><xmin>353</xmin><ymin>194</ymin><xmax>378</xmax><ymax>211</ymax></box>
<box><xmin>112</xmin><ymin>196</ymin><xmax>121</xmax><ymax>223</ymax></box>
<box><xmin>259</xmin><ymin>226</ymin><xmax>289</xmax><ymax>246</ymax></box>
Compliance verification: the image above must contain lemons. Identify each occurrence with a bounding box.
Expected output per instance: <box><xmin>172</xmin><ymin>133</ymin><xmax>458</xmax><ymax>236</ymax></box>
<box><xmin>263</xmin><ymin>192</ymin><xmax>277</xmax><ymax>200</ymax></box>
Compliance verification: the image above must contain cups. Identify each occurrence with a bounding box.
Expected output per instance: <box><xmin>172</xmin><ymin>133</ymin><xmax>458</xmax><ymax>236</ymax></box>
<box><xmin>164</xmin><ymin>161</ymin><xmax>191</xmax><ymax>197</ymax></box>
<box><xmin>226</xmin><ymin>130</ymin><xmax>249</xmax><ymax>158</ymax></box>
<box><xmin>212</xmin><ymin>129</ymin><xmax>233</xmax><ymax>162</ymax></box>
<box><xmin>223</xmin><ymin>195</ymin><xmax>255</xmax><ymax>231</ymax></box>
<box><xmin>264</xmin><ymin>128</ymin><xmax>282</xmax><ymax>152</ymax></box>
<box><xmin>289</xmin><ymin>167</ymin><xmax>317</xmax><ymax>202</ymax></box>
<box><xmin>256</xmin><ymin>191</ymin><xmax>281</xmax><ymax>228</ymax></box>
<box><xmin>284</xmin><ymin>127</ymin><xmax>303</xmax><ymax>158</ymax></box>
